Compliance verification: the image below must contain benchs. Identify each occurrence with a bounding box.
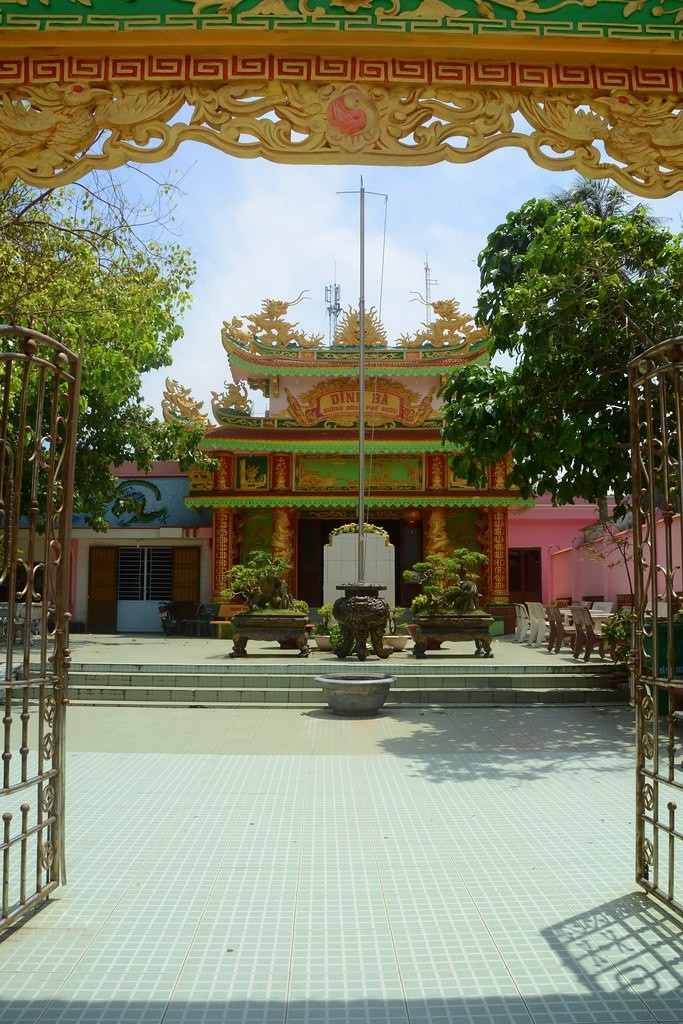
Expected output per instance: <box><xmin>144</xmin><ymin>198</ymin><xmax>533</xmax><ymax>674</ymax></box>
<box><xmin>209</xmin><ymin>604</ymin><xmax>251</xmax><ymax>639</ymax></box>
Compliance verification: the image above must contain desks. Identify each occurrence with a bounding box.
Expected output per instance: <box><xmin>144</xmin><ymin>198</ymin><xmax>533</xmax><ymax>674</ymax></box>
<box><xmin>544</xmin><ymin>607</ymin><xmax>605</xmax><ymax>646</ymax></box>
<box><xmin>590</xmin><ymin>613</ymin><xmax>654</xmax><ymax>651</ymax></box>
<box><xmin>0</xmin><ymin>622</ymin><xmax>37</xmax><ymax>645</ymax></box>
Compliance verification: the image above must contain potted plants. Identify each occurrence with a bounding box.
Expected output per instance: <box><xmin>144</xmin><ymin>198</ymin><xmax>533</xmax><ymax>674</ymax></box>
<box><xmin>216</xmin><ymin>551</ymin><xmax>311</xmax><ymax>658</ymax></box>
<box><xmin>311</xmin><ymin>603</ymin><xmax>342</xmax><ymax>651</ymax></box>
<box><xmin>599</xmin><ymin>607</ymin><xmax>635</xmax><ymax>662</ymax></box>
<box><xmin>403</xmin><ymin>547</ymin><xmax>495</xmax><ymax>659</ymax></box>
<box><xmin>382</xmin><ymin>605</ymin><xmax>412</xmax><ymax>652</ymax></box>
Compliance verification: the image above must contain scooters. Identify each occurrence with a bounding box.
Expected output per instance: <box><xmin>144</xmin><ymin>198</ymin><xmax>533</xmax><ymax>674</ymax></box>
<box><xmin>158</xmin><ymin>599</ymin><xmax>185</xmax><ymax>636</ymax></box>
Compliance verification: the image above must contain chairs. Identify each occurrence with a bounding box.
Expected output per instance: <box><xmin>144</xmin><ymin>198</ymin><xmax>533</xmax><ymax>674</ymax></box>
<box><xmin>0</xmin><ymin>601</ymin><xmax>51</xmax><ymax>647</ymax></box>
<box><xmin>158</xmin><ymin>600</ymin><xmax>220</xmax><ymax>637</ymax></box>
<box><xmin>512</xmin><ymin>593</ymin><xmax>667</xmax><ymax>662</ymax></box>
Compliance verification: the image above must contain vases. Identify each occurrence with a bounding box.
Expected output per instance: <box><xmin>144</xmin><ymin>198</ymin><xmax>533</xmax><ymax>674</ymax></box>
<box><xmin>314</xmin><ymin>673</ymin><xmax>398</xmax><ymax>717</ymax></box>
